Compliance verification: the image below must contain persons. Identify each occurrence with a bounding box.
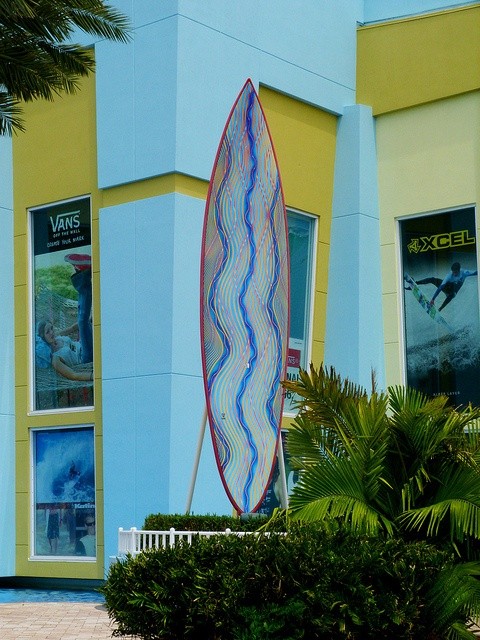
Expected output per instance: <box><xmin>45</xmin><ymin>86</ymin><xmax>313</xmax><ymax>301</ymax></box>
<box><xmin>404</xmin><ymin>262</ymin><xmax>478</xmax><ymax>313</ymax></box>
<box><xmin>63</xmin><ymin>505</ymin><xmax>96</xmax><ymax>556</ymax></box>
<box><xmin>36</xmin><ymin>253</ymin><xmax>94</xmax><ymax>381</ymax></box>
<box><xmin>45</xmin><ymin>506</ymin><xmax>63</xmax><ymax>552</ymax></box>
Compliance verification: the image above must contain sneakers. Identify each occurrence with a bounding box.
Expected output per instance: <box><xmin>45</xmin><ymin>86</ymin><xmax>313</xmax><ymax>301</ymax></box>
<box><xmin>64</xmin><ymin>254</ymin><xmax>91</xmax><ymax>272</ymax></box>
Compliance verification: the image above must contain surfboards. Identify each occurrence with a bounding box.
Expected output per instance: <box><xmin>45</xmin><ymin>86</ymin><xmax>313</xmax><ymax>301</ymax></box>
<box><xmin>405</xmin><ymin>274</ymin><xmax>447</xmax><ymax>327</ymax></box>
<box><xmin>200</xmin><ymin>77</ymin><xmax>290</xmax><ymax>518</ymax></box>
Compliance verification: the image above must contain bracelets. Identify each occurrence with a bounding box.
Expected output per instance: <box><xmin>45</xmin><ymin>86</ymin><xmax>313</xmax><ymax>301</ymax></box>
<box><xmin>90</xmin><ymin>371</ymin><xmax>93</xmax><ymax>381</ymax></box>
